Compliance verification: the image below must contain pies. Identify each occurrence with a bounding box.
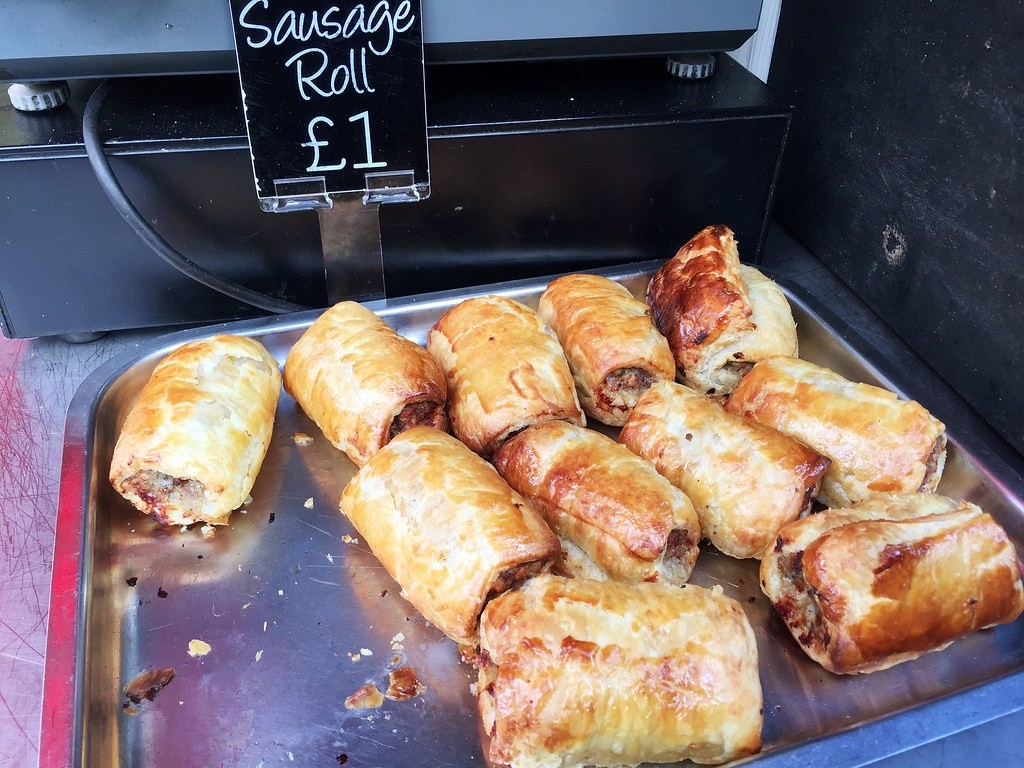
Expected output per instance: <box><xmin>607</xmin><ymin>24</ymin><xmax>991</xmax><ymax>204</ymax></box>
<box><xmin>106</xmin><ymin>228</ymin><xmax>1024</xmax><ymax>768</ymax></box>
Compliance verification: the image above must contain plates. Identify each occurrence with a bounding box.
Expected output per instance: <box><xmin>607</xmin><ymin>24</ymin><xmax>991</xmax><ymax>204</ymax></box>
<box><xmin>41</xmin><ymin>258</ymin><xmax>1022</xmax><ymax>768</ymax></box>
